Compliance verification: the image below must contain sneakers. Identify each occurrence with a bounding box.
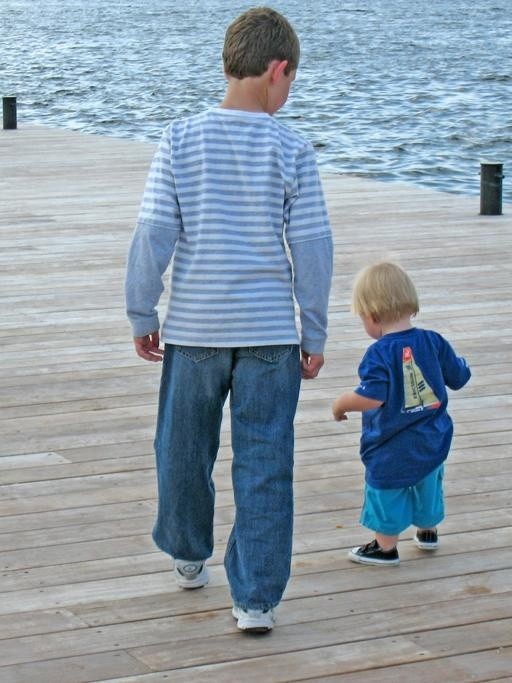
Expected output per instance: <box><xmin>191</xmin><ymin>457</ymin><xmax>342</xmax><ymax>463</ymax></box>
<box><xmin>414</xmin><ymin>528</ymin><xmax>440</xmax><ymax>551</ymax></box>
<box><xmin>348</xmin><ymin>539</ymin><xmax>400</xmax><ymax>567</ymax></box>
<box><xmin>173</xmin><ymin>558</ymin><xmax>210</xmax><ymax>590</ymax></box>
<box><xmin>232</xmin><ymin>605</ymin><xmax>276</xmax><ymax>633</ymax></box>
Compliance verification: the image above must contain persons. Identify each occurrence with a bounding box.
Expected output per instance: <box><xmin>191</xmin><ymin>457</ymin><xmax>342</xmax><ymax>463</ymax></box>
<box><xmin>332</xmin><ymin>261</ymin><xmax>471</xmax><ymax>566</ymax></box>
<box><xmin>124</xmin><ymin>6</ymin><xmax>334</xmax><ymax>633</ymax></box>
